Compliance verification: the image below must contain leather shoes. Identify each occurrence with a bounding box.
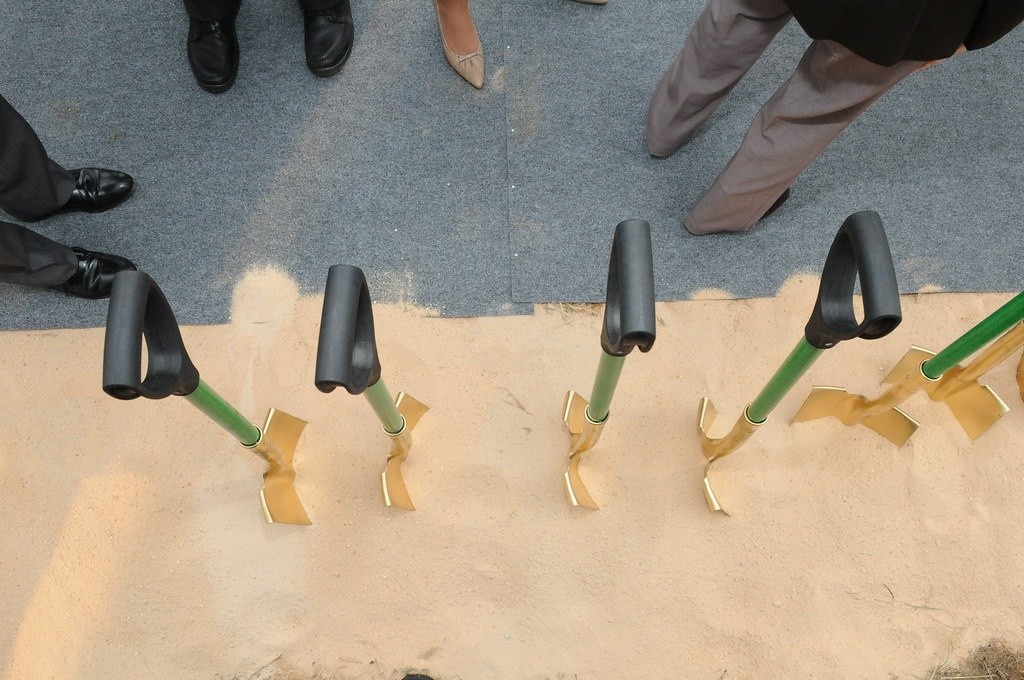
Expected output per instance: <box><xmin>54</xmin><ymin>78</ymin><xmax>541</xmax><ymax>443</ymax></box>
<box><xmin>293</xmin><ymin>0</ymin><xmax>355</xmax><ymax>77</ymax></box>
<box><xmin>27</xmin><ymin>167</ymin><xmax>135</xmax><ymax>222</ymax></box>
<box><xmin>47</xmin><ymin>247</ymin><xmax>138</xmax><ymax>300</ymax></box>
<box><xmin>187</xmin><ymin>3</ymin><xmax>240</xmax><ymax>91</ymax></box>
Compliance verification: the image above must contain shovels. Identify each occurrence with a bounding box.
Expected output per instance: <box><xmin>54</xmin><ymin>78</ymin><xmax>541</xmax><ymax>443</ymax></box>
<box><xmin>881</xmin><ymin>320</ymin><xmax>1024</xmax><ymax>441</ymax></box>
<box><xmin>790</xmin><ymin>290</ymin><xmax>1024</xmax><ymax>448</ymax></box>
<box><xmin>697</xmin><ymin>211</ymin><xmax>902</xmax><ymax>517</ymax></box>
<box><xmin>315</xmin><ymin>264</ymin><xmax>430</xmax><ymax>512</ymax></box>
<box><xmin>102</xmin><ymin>269</ymin><xmax>311</xmax><ymax>524</ymax></box>
<box><xmin>563</xmin><ymin>219</ymin><xmax>657</xmax><ymax>510</ymax></box>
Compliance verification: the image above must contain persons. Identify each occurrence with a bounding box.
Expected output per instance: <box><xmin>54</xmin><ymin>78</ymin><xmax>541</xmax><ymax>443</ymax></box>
<box><xmin>646</xmin><ymin>0</ymin><xmax>1024</xmax><ymax>234</ymax></box>
<box><xmin>436</xmin><ymin>0</ymin><xmax>483</xmax><ymax>89</ymax></box>
<box><xmin>0</xmin><ymin>94</ymin><xmax>137</xmax><ymax>300</ymax></box>
<box><xmin>184</xmin><ymin>0</ymin><xmax>354</xmax><ymax>95</ymax></box>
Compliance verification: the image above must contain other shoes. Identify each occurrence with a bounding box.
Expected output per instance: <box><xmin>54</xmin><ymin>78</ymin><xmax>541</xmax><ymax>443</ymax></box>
<box><xmin>761</xmin><ymin>189</ymin><xmax>791</xmax><ymax>218</ymax></box>
<box><xmin>434</xmin><ymin>0</ymin><xmax>484</xmax><ymax>88</ymax></box>
